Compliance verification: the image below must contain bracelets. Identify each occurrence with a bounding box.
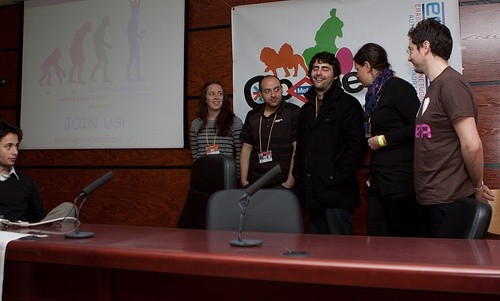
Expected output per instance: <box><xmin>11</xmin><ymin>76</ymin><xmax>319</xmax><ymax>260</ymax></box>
<box><xmin>475</xmin><ymin>181</ymin><xmax>483</xmax><ymax>192</ymax></box>
<box><xmin>378</xmin><ymin>135</ymin><xmax>386</xmax><ymax>147</ymax></box>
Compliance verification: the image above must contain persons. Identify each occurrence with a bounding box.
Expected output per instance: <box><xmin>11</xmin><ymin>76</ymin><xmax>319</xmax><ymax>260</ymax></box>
<box><xmin>408</xmin><ymin>17</ymin><xmax>495</xmax><ymax>238</ymax></box>
<box><xmin>291</xmin><ymin>52</ymin><xmax>368</xmax><ymax>235</ymax></box>
<box><xmin>352</xmin><ymin>43</ymin><xmax>421</xmax><ymax>237</ymax></box>
<box><xmin>190</xmin><ymin>81</ymin><xmax>243</xmax><ymax>180</ymax></box>
<box><xmin>0</xmin><ymin>120</ymin><xmax>45</xmax><ymax>227</ymax></box>
<box><xmin>240</xmin><ymin>75</ymin><xmax>300</xmax><ymax>189</ymax></box>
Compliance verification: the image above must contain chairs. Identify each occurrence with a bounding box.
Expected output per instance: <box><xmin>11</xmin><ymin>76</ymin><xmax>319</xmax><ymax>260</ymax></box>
<box><xmin>208</xmin><ymin>189</ymin><xmax>305</xmax><ymax>234</ymax></box>
<box><xmin>431</xmin><ymin>198</ymin><xmax>491</xmax><ymax>241</ymax></box>
<box><xmin>177</xmin><ymin>155</ymin><xmax>237</xmax><ymax>229</ymax></box>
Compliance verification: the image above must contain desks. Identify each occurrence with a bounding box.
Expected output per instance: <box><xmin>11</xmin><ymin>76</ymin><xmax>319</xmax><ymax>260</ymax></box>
<box><xmin>0</xmin><ymin>224</ymin><xmax>500</xmax><ymax>301</ymax></box>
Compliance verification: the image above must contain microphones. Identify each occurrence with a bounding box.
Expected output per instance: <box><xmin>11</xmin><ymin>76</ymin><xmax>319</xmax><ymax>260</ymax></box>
<box><xmin>239</xmin><ymin>164</ymin><xmax>282</xmax><ymax>202</ymax></box>
<box><xmin>80</xmin><ymin>171</ymin><xmax>114</xmax><ymax>197</ymax></box>
